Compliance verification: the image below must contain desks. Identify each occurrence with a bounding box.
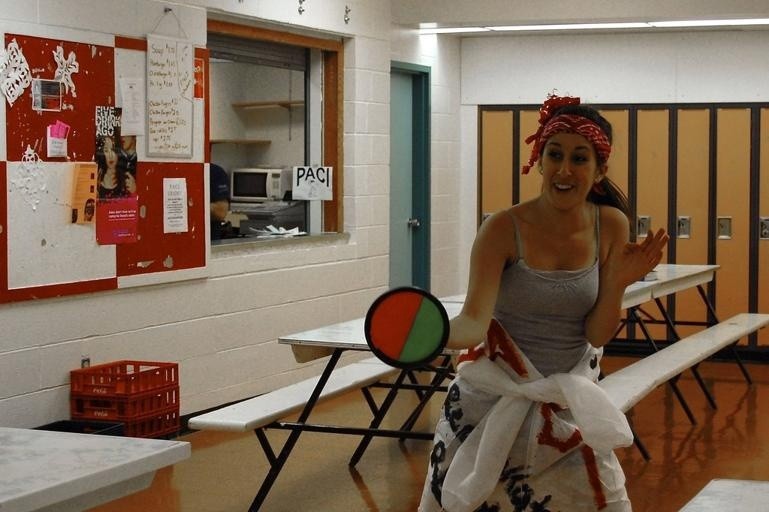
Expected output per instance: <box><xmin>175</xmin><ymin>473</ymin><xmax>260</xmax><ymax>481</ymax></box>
<box><xmin>0</xmin><ymin>422</ymin><xmax>192</xmax><ymax>512</ymax></box>
<box><xmin>241</xmin><ymin>202</ymin><xmax>307</xmax><ymax>237</ymax></box>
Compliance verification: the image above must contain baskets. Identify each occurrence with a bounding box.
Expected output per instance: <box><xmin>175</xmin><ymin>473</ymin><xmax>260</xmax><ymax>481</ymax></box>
<box><xmin>70</xmin><ymin>359</ymin><xmax>181</xmax><ymax>439</ymax></box>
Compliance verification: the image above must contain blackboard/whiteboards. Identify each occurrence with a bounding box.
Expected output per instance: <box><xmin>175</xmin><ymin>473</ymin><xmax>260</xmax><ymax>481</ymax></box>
<box><xmin>146</xmin><ymin>33</ymin><xmax>194</xmax><ymax>158</ymax></box>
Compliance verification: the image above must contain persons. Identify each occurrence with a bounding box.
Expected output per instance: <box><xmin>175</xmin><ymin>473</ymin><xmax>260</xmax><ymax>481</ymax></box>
<box><xmin>417</xmin><ymin>92</ymin><xmax>670</xmax><ymax>512</ymax></box>
<box><xmin>210</xmin><ymin>162</ymin><xmax>231</xmax><ymax>239</ymax></box>
<box><xmin>98</xmin><ymin>135</ymin><xmax>136</xmax><ymax>199</ymax></box>
<box><xmin>116</xmin><ymin>115</ymin><xmax>137</xmax><ymax>174</ymax></box>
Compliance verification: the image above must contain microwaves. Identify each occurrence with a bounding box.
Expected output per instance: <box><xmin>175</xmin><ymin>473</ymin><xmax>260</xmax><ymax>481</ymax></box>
<box><xmin>230</xmin><ymin>167</ymin><xmax>287</xmax><ymax>201</ymax></box>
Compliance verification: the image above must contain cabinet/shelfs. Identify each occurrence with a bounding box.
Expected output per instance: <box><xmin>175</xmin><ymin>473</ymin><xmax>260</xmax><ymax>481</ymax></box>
<box><xmin>209</xmin><ymin>99</ymin><xmax>305</xmax><ymax>145</ymax></box>
